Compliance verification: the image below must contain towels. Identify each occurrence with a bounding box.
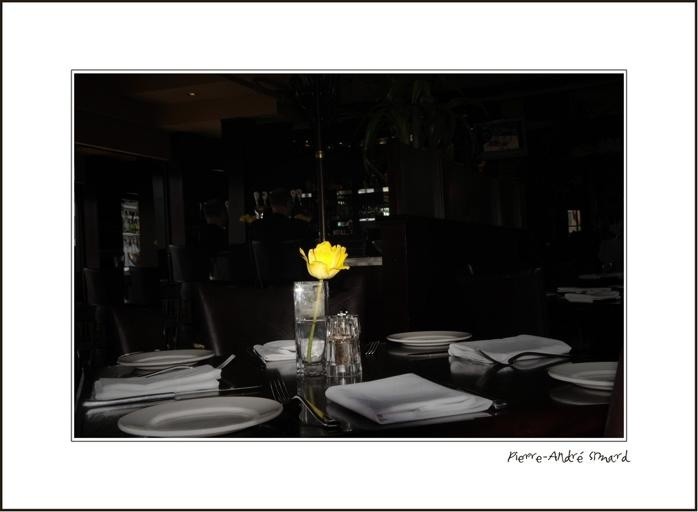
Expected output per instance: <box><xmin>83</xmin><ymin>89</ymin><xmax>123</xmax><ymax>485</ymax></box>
<box><xmin>323</xmin><ymin>370</ymin><xmax>494</xmax><ymax>424</ymax></box>
<box><xmin>92</xmin><ymin>361</ymin><xmax>222</xmax><ymax>397</ymax></box>
<box><xmin>447</xmin><ymin>334</ymin><xmax>572</xmax><ymax>364</ymax></box>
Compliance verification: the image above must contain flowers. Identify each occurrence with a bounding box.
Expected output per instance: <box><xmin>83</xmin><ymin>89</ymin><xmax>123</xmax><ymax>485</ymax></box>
<box><xmin>298</xmin><ymin>242</ymin><xmax>350</xmax><ymax>365</ymax></box>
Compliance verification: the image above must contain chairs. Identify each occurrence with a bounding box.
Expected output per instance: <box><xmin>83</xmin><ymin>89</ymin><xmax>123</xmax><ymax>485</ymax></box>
<box><xmin>196</xmin><ymin>273</ymin><xmax>367</xmax><ymax>357</ymax></box>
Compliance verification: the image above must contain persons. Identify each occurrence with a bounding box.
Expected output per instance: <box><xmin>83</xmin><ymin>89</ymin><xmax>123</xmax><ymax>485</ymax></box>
<box><xmin>179</xmin><ymin>186</ymin><xmax>312</xmax><ymax>300</ymax></box>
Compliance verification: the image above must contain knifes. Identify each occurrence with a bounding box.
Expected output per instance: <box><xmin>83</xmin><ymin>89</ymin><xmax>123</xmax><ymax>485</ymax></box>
<box><xmin>82</xmin><ymin>384</ymin><xmax>263</xmax><ymax>408</ymax></box>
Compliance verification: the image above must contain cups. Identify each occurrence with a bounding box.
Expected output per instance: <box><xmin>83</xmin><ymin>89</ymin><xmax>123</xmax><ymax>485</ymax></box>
<box><xmin>294</xmin><ymin>281</ymin><xmax>365</xmax><ymax>378</ymax></box>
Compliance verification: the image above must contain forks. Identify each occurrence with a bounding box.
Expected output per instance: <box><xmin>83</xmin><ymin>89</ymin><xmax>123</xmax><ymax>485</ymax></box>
<box><xmin>365</xmin><ymin>339</ymin><xmax>385</xmax><ymax>355</ymax></box>
<box><xmin>140</xmin><ymin>353</ymin><xmax>236</xmax><ymax>377</ymax></box>
<box><xmin>477</xmin><ymin>347</ymin><xmax>577</xmax><ymax>367</ymax></box>
<box><xmin>262</xmin><ymin>370</ymin><xmax>339</xmax><ymax>430</ymax></box>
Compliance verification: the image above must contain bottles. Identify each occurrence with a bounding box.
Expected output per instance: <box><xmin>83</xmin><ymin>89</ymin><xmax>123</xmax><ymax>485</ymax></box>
<box><xmin>332</xmin><ymin>177</ymin><xmax>390</xmax><ymax>246</ymax></box>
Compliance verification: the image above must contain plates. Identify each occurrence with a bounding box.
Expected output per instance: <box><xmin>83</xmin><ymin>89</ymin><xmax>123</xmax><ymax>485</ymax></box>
<box><xmin>548</xmin><ymin>361</ymin><xmax>621</xmax><ymax>390</ymax></box>
<box><xmin>117</xmin><ymin>395</ymin><xmax>283</xmax><ymax>435</ymax></box>
<box><xmin>385</xmin><ymin>330</ymin><xmax>473</xmax><ymax>347</ymax></box>
<box><xmin>119</xmin><ymin>350</ymin><xmax>214</xmax><ymax>371</ymax></box>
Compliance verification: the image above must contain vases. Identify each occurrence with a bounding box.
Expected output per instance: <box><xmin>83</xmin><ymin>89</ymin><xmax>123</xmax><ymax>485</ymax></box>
<box><xmin>293</xmin><ymin>281</ymin><xmax>329</xmax><ymax>365</ymax></box>
